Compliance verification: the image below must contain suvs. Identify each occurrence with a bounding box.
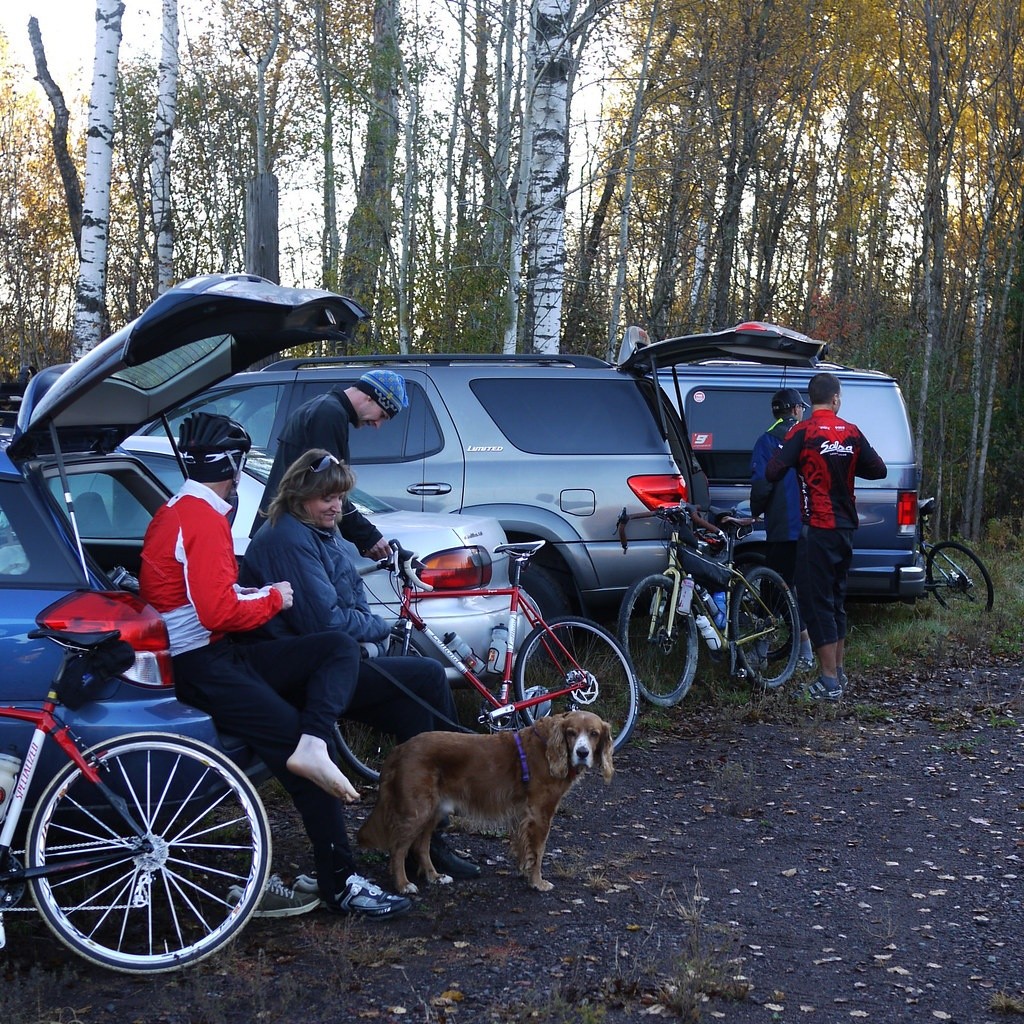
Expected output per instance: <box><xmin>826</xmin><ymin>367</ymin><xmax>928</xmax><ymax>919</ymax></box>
<box><xmin>139</xmin><ymin>319</ymin><xmax>834</xmax><ymax>669</ymax></box>
<box><xmin>614</xmin><ymin>324</ymin><xmax>929</xmax><ymax>606</ymax></box>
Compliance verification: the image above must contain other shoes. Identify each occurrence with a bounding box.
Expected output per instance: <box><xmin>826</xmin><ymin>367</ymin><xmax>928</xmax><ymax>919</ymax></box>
<box><xmin>406</xmin><ymin>834</ymin><xmax>483</xmax><ymax>879</ymax></box>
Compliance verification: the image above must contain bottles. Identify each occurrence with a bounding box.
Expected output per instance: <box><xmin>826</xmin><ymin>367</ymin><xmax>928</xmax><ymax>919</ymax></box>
<box><xmin>487</xmin><ymin>623</ymin><xmax>508</xmax><ymax>674</ymax></box>
<box><xmin>675</xmin><ymin>574</ymin><xmax>694</xmax><ymax>615</ymax></box>
<box><xmin>696</xmin><ymin>614</ymin><xmax>721</xmax><ymax>650</ymax></box>
<box><xmin>695</xmin><ymin>584</ymin><xmax>719</xmax><ymax>616</ymax></box>
<box><xmin>443</xmin><ymin>631</ymin><xmax>486</xmax><ymax>677</ymax></box>
<box><xmin>650</xmin><ymin>588</ymin><xmax>667</xmax><ymax>620</ymax></box>
<box><xmin>713</xmin><ymin>586</ymin><xmax>727</xmax><ymax>629</ymax></box>
<box><xmin>0</xmin><ymin>744</ymin><xmax>23</xmax><ymax>826</ymax></box>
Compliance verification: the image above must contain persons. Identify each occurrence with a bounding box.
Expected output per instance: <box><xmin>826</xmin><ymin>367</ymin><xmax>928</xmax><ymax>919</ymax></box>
<box><xmin>250</xmin><ymin>368</ymin><xmax>410</xmax><ymax>559</ymax></box>
<box><xmin>238</xmin><ymin>447</ymin><xmax>483</xmax><ymax>881</ymax></box>
<box><xmin>765</xmin><ymin>373</ymin><xmax>889</xmax><ymax>700</ymax></box>
<box><xmin>137</xmin><ymin>412</ymin><xmax>411</xmax><ymax>918</ymax></box>
<box><xmin>748</xmin><ymin>388</ymin><xmax>811</xmax><ymax>674</ymax></box>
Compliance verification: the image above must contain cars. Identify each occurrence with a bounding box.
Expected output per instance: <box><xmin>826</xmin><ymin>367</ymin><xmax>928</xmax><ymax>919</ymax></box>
<box><xmin>1</xmin><ymin>425</ymin><xmax>546</xmax><ymax>686</ymax></box>
<box><xmin>0</xmin><ymin>270</ymin><xmax>371</xmax><ymax>922</ymax></box>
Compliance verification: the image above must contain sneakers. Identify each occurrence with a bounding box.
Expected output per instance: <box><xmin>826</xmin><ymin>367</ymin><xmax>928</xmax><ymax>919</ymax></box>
<box><xmin>790</xmin><ymin>682</ymin><xmax>844</xmax><ymax>700</ymax></box>
<box><xmin>795</xmin><ymin>655</ymin><xmax>816</xmax><ymax>671</ymax></box>
<box><xmin>225</xmin><ymin>873</ymin><xmax>322</xmax><ymax>917</ymax></box>
<box><xmin>798</xmin><ymin>673</ymin><xmax>849</xmax><ymax>691</ymax></box>
<box><xmin>323</xmin><ymin>872</ymin><xmax>411</xmax><ymax>920</ymax></box>
<box><xmin>291</xmin><ymin>874</ymin><xmax>327</xmax><ymax>906</ymax></box>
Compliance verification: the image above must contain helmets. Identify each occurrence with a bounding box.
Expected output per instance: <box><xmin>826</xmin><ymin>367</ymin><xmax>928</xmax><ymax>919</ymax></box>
<box><xmin>178</xmin><ymin>410</ymin><xmax>253</xmax><ymax>483</ymax></box>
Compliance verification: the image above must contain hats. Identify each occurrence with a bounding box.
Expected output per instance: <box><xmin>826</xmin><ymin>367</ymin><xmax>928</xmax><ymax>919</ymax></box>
<box><xmin>770</xmin><ymin>388</ymin><xmax>811</xmax><ymax>410</ymax></box>
<box><xmin>356</xmin><ymin>370</ymin><xmax>408</xmax><ymax>420</ymax></box>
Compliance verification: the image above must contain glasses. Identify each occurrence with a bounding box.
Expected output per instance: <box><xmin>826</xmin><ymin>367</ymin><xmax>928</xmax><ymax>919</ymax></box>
<box><xmin>791</xmin><ymin>404</ymin><xmax>806</xmax><ymax>412</ymax></box>
<box><xmin>304</xmin><ymin>455</ymin><xmax>339</xmax><ymax>481</ymax></box>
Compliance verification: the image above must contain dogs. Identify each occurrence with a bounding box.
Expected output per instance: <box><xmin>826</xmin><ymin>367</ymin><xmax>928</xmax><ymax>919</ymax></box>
<box><xmin>357</xmin><ymin>709</ymin><xmax>615</xmax><ymax>896</ymax></box>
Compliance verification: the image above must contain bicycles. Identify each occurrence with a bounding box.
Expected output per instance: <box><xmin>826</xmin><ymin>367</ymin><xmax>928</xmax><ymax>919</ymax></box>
<box><xmin>0</xmin><ymin>628</ymin><xmax>273</xmax><ymax>975</ymax></box>
<box><xmin>609</xmin><ymin>499</ymin><xmax>801</xmax><ymax>710</ymax></box>
<box><xmin>331</xmin><ymin>536</ymin><xmax>643</xmax><ymax>784</ymax></box>
<box><xmin>916</xmin><ymin>496</ymin><xmax>995</xmax><ymax>615</ymax></box>
